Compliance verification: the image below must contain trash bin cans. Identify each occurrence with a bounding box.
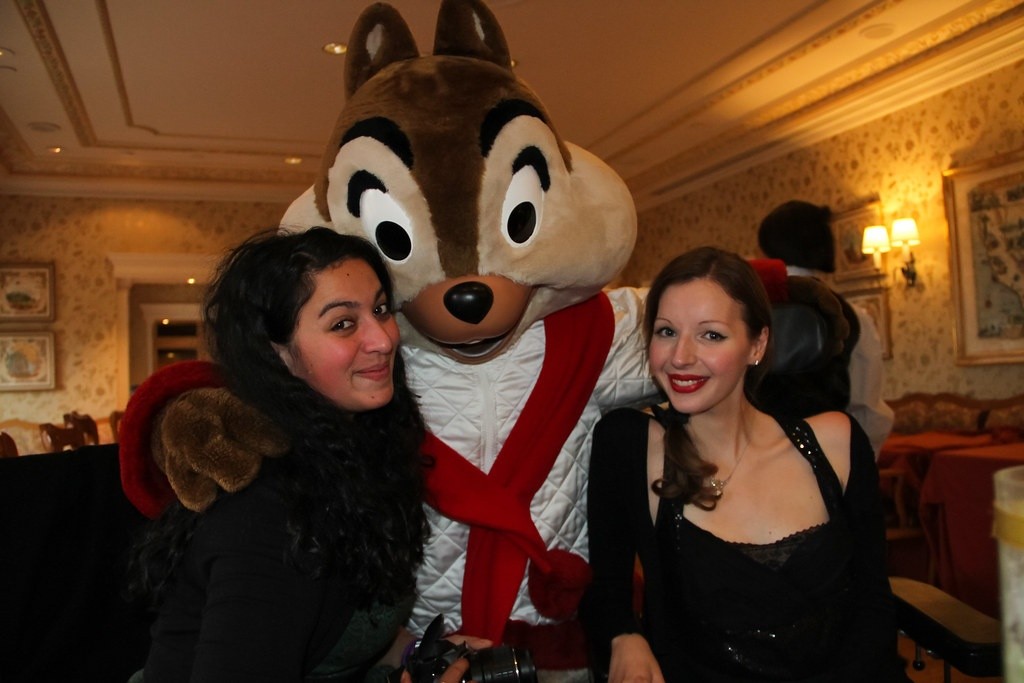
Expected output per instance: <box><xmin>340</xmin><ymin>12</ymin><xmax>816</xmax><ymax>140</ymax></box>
<box><xmin>991</xmin><ymin>466</ymin><xmax>1024</xmax><ymax>683</ymax></box>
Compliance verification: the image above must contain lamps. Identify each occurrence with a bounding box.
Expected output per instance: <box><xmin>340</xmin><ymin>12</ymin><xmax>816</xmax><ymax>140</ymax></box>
<box><xmin>862</xmin><ymin>218</ymin><xmax>920</xmax><ymax>289</ymax></box>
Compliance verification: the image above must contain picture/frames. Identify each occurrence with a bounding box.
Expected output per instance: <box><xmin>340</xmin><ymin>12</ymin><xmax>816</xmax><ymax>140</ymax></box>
<box><xmin>942</xmin><ymin>150</ymin><xmax>1024</xmax><ymax>367</ymax></box>
<box><xmin>0</xmin><ymin>331</ymin><xmax>56</xmax><ymax>392</ymax></box>
<box><xmin>0</xmin><ymin>262</ymin><xmax>55</xmax><ymax>322</ymax></box>
<box><xmin>829</xmin><ymin>200</ymin><xmax>882</xmax><ymax>284</ymax></box>
<box><xmin>841</xmin><ymin>286</ymin><xmax>893</xmax><ymax>362</ymax></box>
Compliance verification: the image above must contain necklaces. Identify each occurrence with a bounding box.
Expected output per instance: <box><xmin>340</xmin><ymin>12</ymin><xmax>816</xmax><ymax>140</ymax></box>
<box><xmin>683</xmin><ymin>412</ymin><xmax>756</xmax><ymax>498</ymax></box>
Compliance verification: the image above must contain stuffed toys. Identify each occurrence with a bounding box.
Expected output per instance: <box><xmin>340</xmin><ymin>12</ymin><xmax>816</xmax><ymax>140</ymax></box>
<box><xmin>118</xmin><ymin>0</ymin><xmax>861</xmax><ymax>683</ymax></box>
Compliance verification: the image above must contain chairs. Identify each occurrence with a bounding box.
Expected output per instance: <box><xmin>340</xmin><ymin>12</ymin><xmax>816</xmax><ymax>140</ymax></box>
<box><xmin>0</xmin><ymin>411</ymin><xmax>124</xmax><ymax>459</ymax></box>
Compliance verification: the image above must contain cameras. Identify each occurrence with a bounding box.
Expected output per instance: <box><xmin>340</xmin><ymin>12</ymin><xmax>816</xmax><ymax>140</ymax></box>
<box><xmin>406</xmin><ymin>613</ymin><xmax>539</xmax><ymax>683</ymax></box>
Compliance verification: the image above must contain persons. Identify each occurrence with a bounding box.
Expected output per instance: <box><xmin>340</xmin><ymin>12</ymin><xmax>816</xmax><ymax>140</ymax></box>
<box><xmin>739</xmin><ymin>201</ymin><xmax>898</xmax><ymax>465</ymax></box>
<box><xmin>126</xmin><ymin>226</ymin><xmax>475</xmax><ymax>683</ymax></box>
<box><xmin>586</xmin><ymin>244</ymin><xmax>898</xmax><ymax>683</ymax></box>
